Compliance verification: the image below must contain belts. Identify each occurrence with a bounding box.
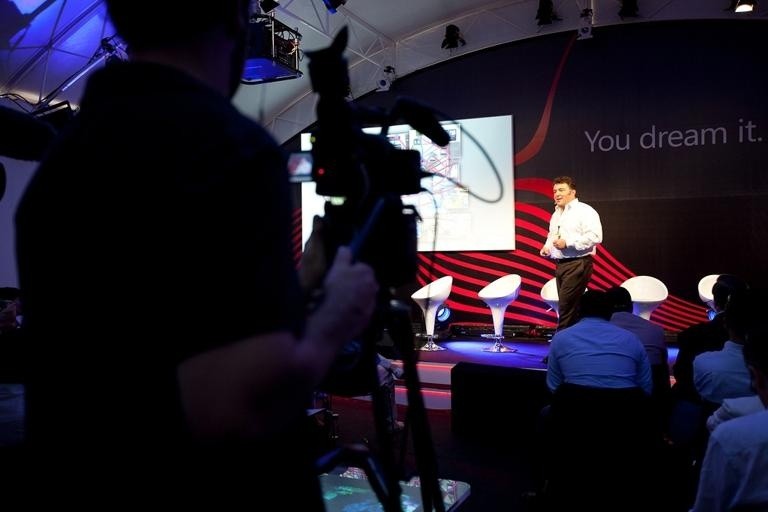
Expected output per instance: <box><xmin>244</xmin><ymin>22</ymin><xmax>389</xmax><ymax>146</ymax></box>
<box><xmin>558</xmin><ymin>258</ymin><xmax>580</xmax><ymax>264</ymax></box>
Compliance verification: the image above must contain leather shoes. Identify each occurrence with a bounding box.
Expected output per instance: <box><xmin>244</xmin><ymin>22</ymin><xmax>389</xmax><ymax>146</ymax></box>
<box><xmin>378</xmin><ymin>418</ymin><xmax>405</xmax><ymax>432</ymax></box>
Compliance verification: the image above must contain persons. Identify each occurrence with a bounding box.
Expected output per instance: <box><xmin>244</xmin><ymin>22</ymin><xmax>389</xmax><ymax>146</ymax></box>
<box><xmin>607</xmin><ymin>287</ymin><xmax>670</xmax><ymax>382</ymax></box>
<box><xmin>705</xmin><ymin>368</ymin><xmax>768</xmax><ymax>433</ymax></box>
<box><xmin>689</xmin><ymin>332</ymin><xmax>767</xmax><ymax>511</ymax></box>
<box><xmin>13</xmin><ymin>0</ymin><xmax>380</xmax><ymax>512</ymax></box>
<box><xmin>546</xmin><ymin>288</ymin><xmax>656</xmax><ymax>399</ymax></box>
<box><xmin>692</xmin><ymin>296</ymin><xmax>756</xmax><ymax>406</ymax></box>
<box><xmin>539</xmin><ymin>176</ymin><xmax>604</xmax><ymax>360</ymax></box>
<box><xmin>673</xmin><ymin>274</ymin><xmax>745</xmax><ymax>362</ymax></box>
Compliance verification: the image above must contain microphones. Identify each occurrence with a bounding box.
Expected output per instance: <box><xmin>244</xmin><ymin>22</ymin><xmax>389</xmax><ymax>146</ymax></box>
<box><xmin>555</xmin><ymin>225</ymin><xmax>562</xmax><ymax>240</ymax></box>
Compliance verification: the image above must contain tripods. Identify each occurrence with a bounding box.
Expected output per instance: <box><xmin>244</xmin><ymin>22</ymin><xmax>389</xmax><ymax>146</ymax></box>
<box><xmin>310</xmin><ymin>198</ymin><xmax>449</xmax><ymax>511</ymax></box>
